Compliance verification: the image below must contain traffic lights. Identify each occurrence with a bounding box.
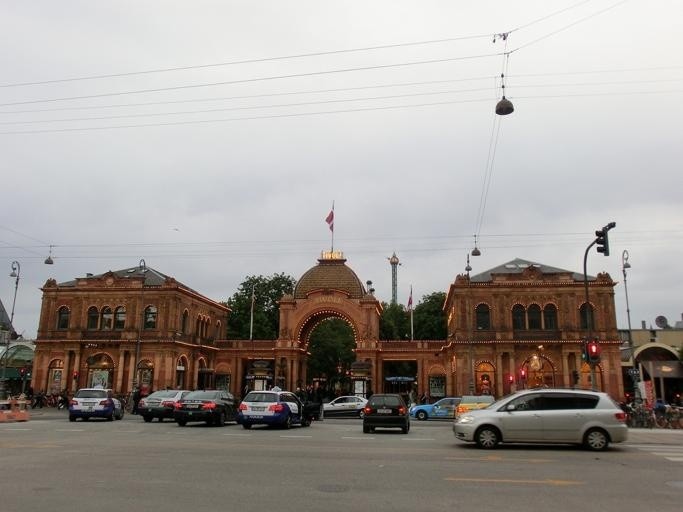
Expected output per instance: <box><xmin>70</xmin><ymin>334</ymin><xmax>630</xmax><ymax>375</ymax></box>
<box><xmin>594</xmin><ymin>226</ymin><xmax>609</xmax><ymax>257</ymax></box>
<box><xmin>20</xmin><ymin>368</ymin><xmax>25</xmax><ymax>378</ymax></box>
<box><xmin>586</xmin><ymin>335</ymin><xmax>600</xmax><ymax>364</ymax></box>
<box><xmin>579</xmin><ymin>335</ymin><xmax>588</xmax><ymax>362</ymax></box>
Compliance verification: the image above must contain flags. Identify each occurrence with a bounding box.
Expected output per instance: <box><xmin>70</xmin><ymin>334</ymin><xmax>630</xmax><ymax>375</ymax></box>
<box><xmin>407</xmin><ymin>286</ymin><xmax>411</xmax><ymax>306</ymax></box>
<box><xmin>325</xmin><ymin>205</ymin><xmax>334</xmax><ymax>232</ymax></box>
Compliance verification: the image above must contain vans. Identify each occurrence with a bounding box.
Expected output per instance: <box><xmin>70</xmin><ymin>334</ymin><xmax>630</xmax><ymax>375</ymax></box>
<box><xmin>450</xmin><ymin>384</ymin><xmax>630</xmax><ymax>453</ymax></box>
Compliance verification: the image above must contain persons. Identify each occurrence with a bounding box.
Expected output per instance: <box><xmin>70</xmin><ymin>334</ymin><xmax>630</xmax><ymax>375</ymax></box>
<box><xmin>420</xmin><ymin>390</ymin><xmax>429</xmax><ymax>403</ymax></box>
<box><xmin>31</xmin><ymin>386</ymin><xmax>44</xmax><ymax>409</ymax></box>
<box><xmin>616</xmin><ymin>396</ymin><xmax>683</xmax><ymax>429</ymax></box>
<box><xmin>130</xmin><ymin>385</ymin><xmax>140</xmax><ymax>416</ymax></box>
<box><xmin>407</xmin><ymin>388</ymin><xmax>415</xmax><ymax>408</ymax></box>
<box><xmin>58</xmin><ymin>387</ymin><xmax>71</xmax><ymax>411</ymax></box>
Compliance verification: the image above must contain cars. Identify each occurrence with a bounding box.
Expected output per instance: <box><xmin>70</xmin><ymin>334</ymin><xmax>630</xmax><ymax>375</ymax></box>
<box><xmin>137</xmin><ymin>388</ymin><xmax>192</xmax><ymax>424</ymax></box>
<box><xmin>322</xmin><ymin>394</ymin><xmax>369</xmax><ymax>420</ymax></box>
<box><xmin>453</xmin><ymin>394</ymin><xmax>495</xmax><ymax>422</ymax></box>
<box><xmin>407</xmin><ymin>396</ymin><xmax>462</xmax><ymax>422</ymax></box>
<box><xmin>66</xmin><ymin>387</ymin><xmax>125</xmax><ymax>421</ymax></box>
<box><xmin>234</xmin><ymin>389</ymin><xmax>322</xmax><ymax>432</ymax></box>
<box><xmin>170</xmin><ymin>387</ymin><xmax>241</xmax><ymax>427</ymax></box>
<box><xmin>361</xmin><ymin>392</ymin><xmax>410</xmax><ymax>436</ymax></box>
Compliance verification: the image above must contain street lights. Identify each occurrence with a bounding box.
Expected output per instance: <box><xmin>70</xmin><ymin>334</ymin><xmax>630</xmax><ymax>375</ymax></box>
<box><xmin>619</xmin><ymin>249</ymin><xmax>642</xmax><ymax>403</ymax></box>
<box><xmin>0</xmin><ymin>260</ymin><xmax>21</xmax><ymax>410</ymax></box>
